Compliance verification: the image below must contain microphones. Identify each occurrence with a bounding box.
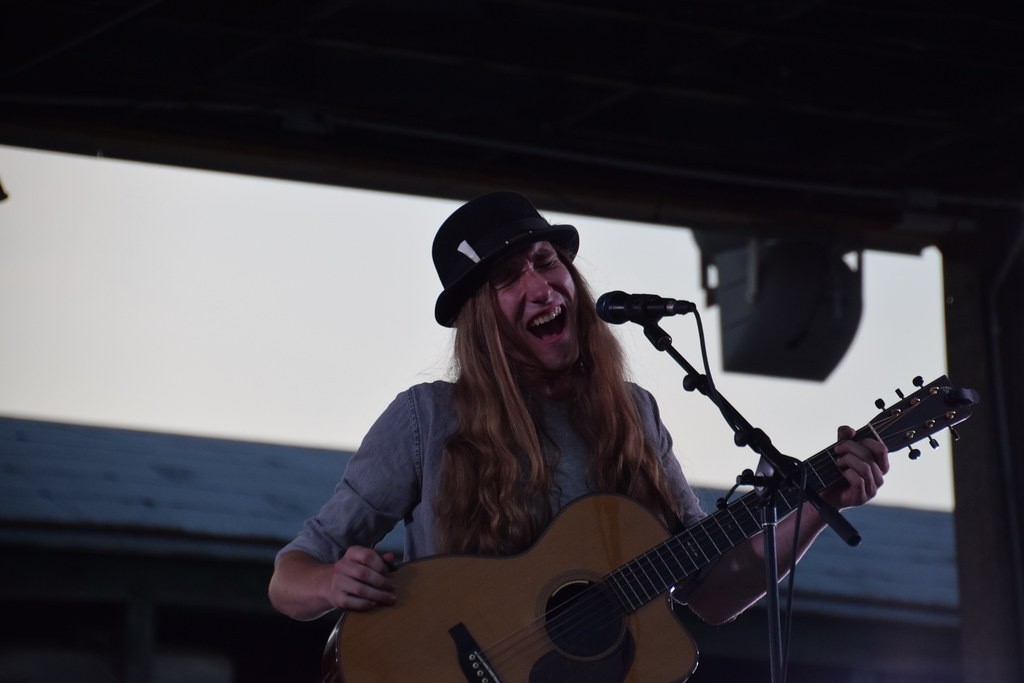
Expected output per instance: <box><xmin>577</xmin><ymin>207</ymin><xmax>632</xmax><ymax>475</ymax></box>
<box><xmin>596</xmin><ymin>290</ymin><xmax>695</xmax><ymax>325</ymax></box>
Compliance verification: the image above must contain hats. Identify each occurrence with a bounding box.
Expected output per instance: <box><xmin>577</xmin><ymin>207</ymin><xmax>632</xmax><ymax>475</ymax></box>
<box><xmin>429</xmin><ymin>192</ymin><xmax>580</xmax><ymax>326</ymax></box>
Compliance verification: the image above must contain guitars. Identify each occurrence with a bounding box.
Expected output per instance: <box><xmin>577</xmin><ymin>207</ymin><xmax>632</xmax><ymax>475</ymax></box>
<box><xmin>321</xmin><ymin>372</ymin><xmax>977</xmax><ymax>683</ymax></box>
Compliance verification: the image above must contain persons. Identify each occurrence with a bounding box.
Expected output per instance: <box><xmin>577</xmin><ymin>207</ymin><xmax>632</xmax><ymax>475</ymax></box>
<box><xmin>266</xmin><ymin>191</ymin><xmax>890</xmax><ymax>683</ymax></box>
